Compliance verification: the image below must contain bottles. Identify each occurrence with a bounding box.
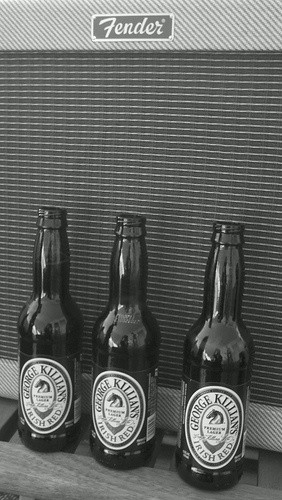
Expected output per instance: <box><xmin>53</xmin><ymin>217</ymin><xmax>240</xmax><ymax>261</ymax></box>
<box><xmin>174</xmin><ymin>222</ymin><xmax>254</xmax><ymax>491</ymax></box>
<box><xmin>17</xmin><ymin>206</ymin><xmax>84</xmax><ymax>452</ymax></box>
<box><xmin>90</xmin><ymin>214</ymin><xmax>162</xmax><ymax>467</ymax></box>
